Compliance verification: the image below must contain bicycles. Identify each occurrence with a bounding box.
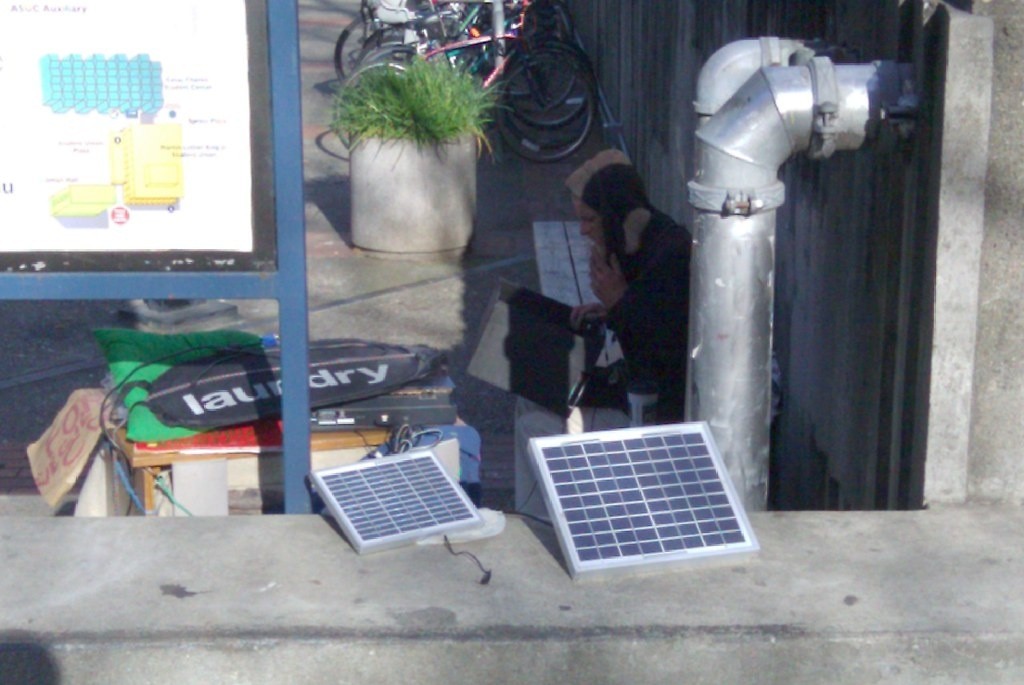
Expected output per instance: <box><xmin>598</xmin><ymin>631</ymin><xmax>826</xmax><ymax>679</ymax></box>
<box><xmin>335</xmin><ymin>0</ymin><xmax>600</xmax><ymax>164</ymax></box>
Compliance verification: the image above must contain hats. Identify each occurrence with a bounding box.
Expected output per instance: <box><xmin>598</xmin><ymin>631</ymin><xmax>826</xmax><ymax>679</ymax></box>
<box><xmin>566</xmin><ymin>148</ymin><xmax>634</xmax><ymax>219</ymax></box>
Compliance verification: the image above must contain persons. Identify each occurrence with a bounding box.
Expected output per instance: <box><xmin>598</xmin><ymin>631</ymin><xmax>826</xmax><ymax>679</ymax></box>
<box><xmin>510</xmin><ymin>146</ymin><xmax>696</xmax><ymax>524</ymax></box>
